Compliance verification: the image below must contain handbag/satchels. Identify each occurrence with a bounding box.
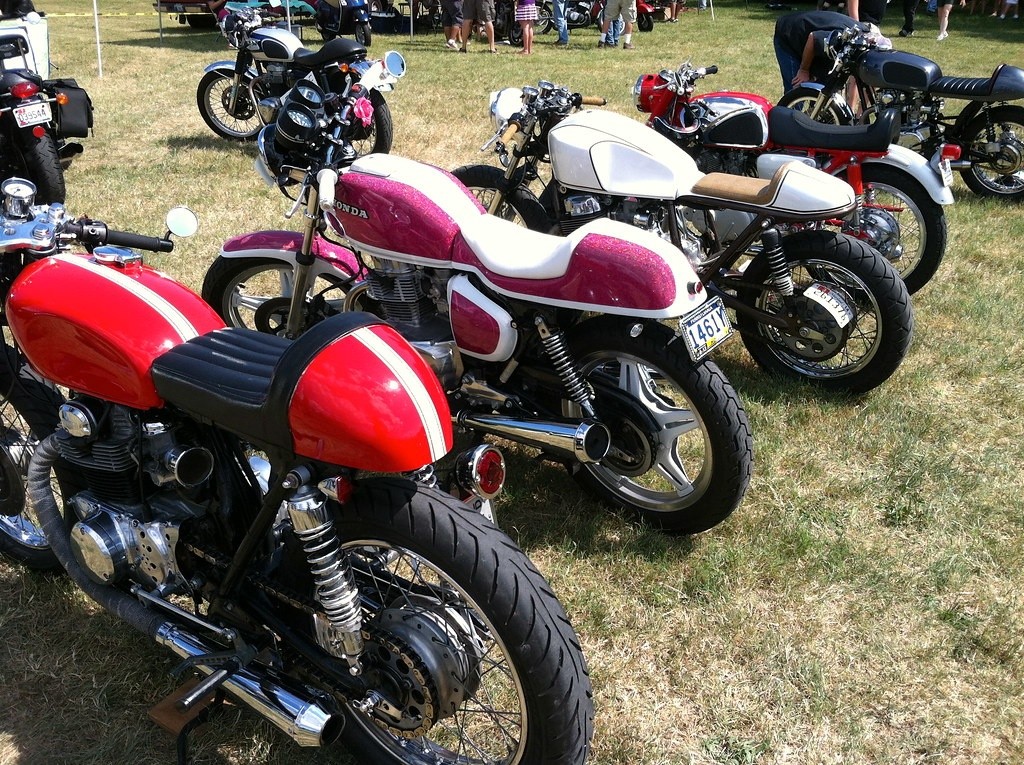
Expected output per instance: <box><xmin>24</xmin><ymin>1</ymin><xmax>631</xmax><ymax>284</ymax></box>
<box><xmin>46</xmin><ymin>78</ymin><xmax>95</xmax><ymax>139</ymax></box>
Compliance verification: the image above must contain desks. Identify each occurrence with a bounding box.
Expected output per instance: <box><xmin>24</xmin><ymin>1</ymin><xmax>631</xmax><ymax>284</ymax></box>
<box><xmin>426</xmin><ymin>4</ymin><xmax>443</xmax><ymax>37</ymax></box>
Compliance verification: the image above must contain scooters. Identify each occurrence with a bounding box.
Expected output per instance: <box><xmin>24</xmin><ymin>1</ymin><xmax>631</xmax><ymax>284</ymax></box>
<box><xmin>433</xmin><ymin>0</ymin><xmax>655</xmax><ymax>47</ymax></box>
<box><xmin>314</xmin><ymin>0</ymin><xmax>372</xmax><ymax>48</ymax></box>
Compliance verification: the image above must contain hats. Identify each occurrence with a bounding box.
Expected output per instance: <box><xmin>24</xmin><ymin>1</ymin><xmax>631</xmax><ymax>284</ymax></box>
<box><xmin>864</xmin><ymin>21</ymin><xmax>893</xmax><ymax>51</ymax></box>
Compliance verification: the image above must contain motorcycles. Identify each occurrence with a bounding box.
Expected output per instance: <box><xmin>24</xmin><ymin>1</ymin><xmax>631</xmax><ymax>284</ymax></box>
<box><xmin>197</xmin><ymin>0</ymin><xmax>393</xmax><ymax>169</ymax></box>
<box><xmin>201</xmin><ymin>33</ymin><xmax>751</xmax><ymax>533</ymax></box>
<box><xmin>449</xmin><ymin>79</ymin><xmax>914</xmax><ymax>399</ymax></box>
<box><xmin>0</xmin><ymin>176</ymin><xmax>595</xmax><ymax>765</ymax></box>
<box><xmin>776</xmin><ymin>23</ymin><xmax>1024</xmax><ymax>204</ymax></box>
<box><xmin>631</xmin><ymin>56</ymin><xmax>961</xmax><ymax>297</ymax></box>
<box><xmin>0</xmin><ymin>0</ymin><xmax>83</xmax><ymax>209</ymax></box>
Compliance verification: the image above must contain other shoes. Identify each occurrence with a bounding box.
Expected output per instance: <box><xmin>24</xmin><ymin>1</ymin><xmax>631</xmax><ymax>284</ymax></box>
<box><xmin>445</xmin><ymin>40</ymin><xmax>458</xmax><ymax>49</ymax></box>
<box><xmin>996</xmin><ymin>16</ymin><xmax>1006</xmax><ymax>21</ymax></box>
<box><xmin>490</xmin><ymin>49</ymin><xmax>498</xmax><ymax>55</ymax></box>
<box><xmin>667</xmin><ymin>18</ymin><xmax>678</xmax><ymax>23</ymax></box>
<box><xmin>623</xmin><ymin>42</ymin><xmax>636</xmax><ymax>50</ymax></box>
<box><xmin>553</xmin><ymin>39</ymin><xmax>568</xmax><ymax>46</ymax></box>
<box><xmin>989</xmin><ymin>13</ymin><xmax>998</xmax><ymax>17</ymax></box>
<box><xmin>598</xmin><ymin>41</ymin><xmax>605</xmax><ymax>50</ymax></box>
<box><xmin>937</xmin><ymin>31</ymin><xmax>948</xmax><ymax>42</ymax></box>
<box><xmin>899</xmin><ymin>30</ymin><xmax>912</xmax><ymax>38</ymax></box>
<box><xmin>459</xmin><ymin>48</ymin><xmax>466</xmax><ymax>53</ymax></box>
<box><xmin>1012</xmin><ymin>17</ymin><xmax>1018</xmax><ymax>23</ymax></box>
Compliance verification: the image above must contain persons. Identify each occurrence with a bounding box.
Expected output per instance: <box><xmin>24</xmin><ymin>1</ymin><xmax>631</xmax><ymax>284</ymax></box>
<box><xmin>899</xmin><ymin>0</ymin><xmax>1024</xmax><ymax>41</ymax></box>
<box><xmin>774</xmin><ymin>11</ymin><xmax>891</xmax><ymax>117</ymax></box>
<box><xmin>406</xmin><ymin>0</ymin><xmax>707</xmax><ymax>54</ymax></box>
<box><xmin>206</xmin><ymin>0</ymin><xmax>228</xmax><ymax>22</ymax></box>
<box><xmin>841</xmin><ymin>0</ymin><xmax>890</xmax><ymax>125</ymax></box>
